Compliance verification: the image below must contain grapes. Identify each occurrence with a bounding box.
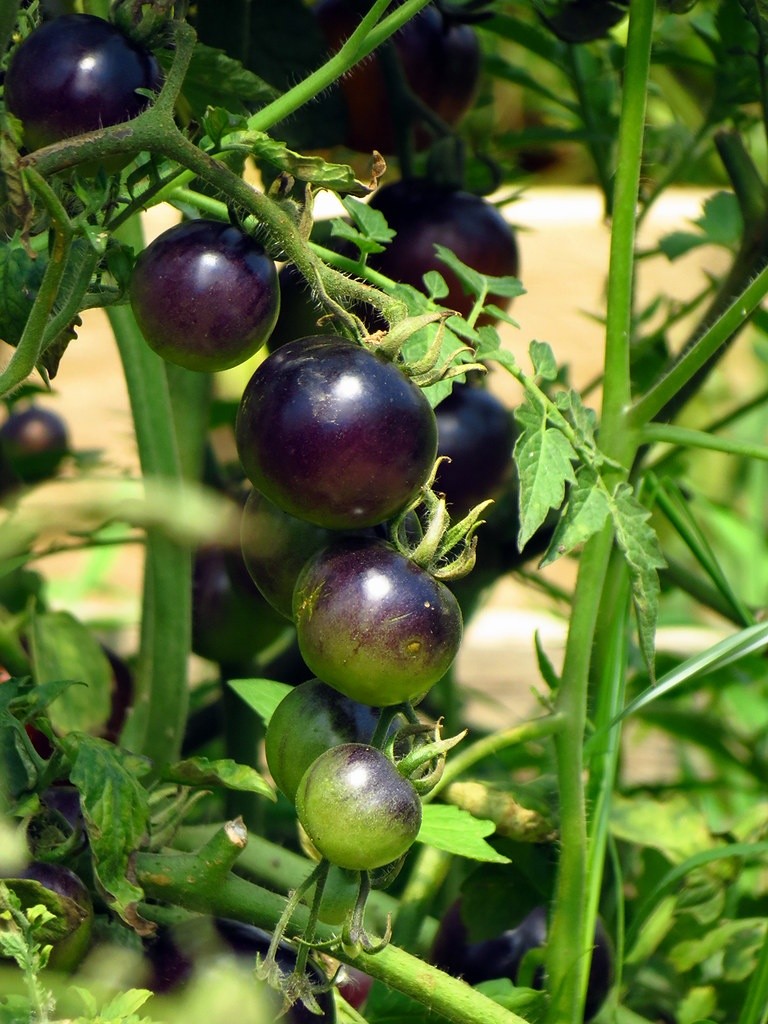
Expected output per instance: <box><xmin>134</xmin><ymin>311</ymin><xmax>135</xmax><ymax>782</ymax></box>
<box><xmin>0</xmin><ymin>0</ymin><xmax>639</xmax><ymax>1024</ymax></box>
